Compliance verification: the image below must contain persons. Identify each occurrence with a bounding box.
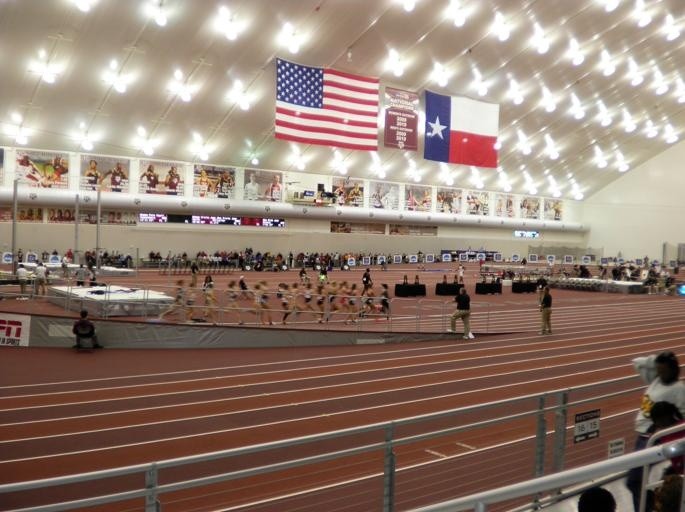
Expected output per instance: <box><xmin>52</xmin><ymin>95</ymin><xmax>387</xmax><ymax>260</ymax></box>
<box><xmin>19</xmin><ymin>207</ymin><xmax>137</xmax><ymax>224</ymax></box>
<box><xmin>333</xmin><ymin>180</ymin><xmax>561</xmax><ymax>220</ymax></box>
<box><xmin>558</xmin><ymin>254</ymin><xmax>681</xmax><ymax>297</ymax></box>
<box><xmin>14</xmin><ymin>154</ymin><xmax>283</xmax><ymax>203</ymax></box>
<box><xmin>17</xmin><ymin>245</ymin><xmax>552</xmax><ymax>349</ymax></box>
<box><xmin>576</xmin><ymin>353</ymin><xmax>685</xmax><ymax>512</ymax></box>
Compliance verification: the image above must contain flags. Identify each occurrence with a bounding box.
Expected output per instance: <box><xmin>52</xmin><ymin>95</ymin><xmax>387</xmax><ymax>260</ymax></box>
<box><xmin>424</xmin><ymin>88</ymin><xmax>500</xmax><ymax>167</ymax></box>
<box><xmin>274</xmin><ymin>57</ymin><xmax>381</xmax><ymax>151</ymax></box>
